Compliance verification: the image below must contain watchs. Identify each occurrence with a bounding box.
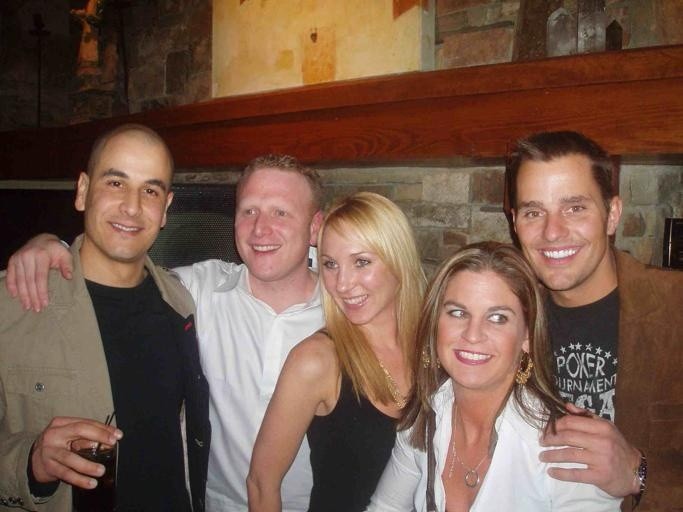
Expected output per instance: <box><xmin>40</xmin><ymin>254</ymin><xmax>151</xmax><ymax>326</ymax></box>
<box><xmin>637</xmin><ymin>455</ymin><xmax>646</xmax><ymax>493</ymax></box>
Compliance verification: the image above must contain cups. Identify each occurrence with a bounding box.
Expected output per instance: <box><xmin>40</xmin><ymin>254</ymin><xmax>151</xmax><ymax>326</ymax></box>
<box><xmin>71</xmin><ymin>438</ymin><xmax>118</xmax><ymax>511</ymax></box>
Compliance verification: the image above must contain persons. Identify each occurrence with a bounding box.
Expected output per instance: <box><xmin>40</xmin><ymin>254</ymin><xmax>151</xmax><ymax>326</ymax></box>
<box><xmin>1</xmin><ymin>152</ymin><xmax>328</xmax><ymax>512</ymax></box>
<box><xmin>0</xmin><ymin>121</ymin><xmax>210</xmax><ymax>512</ymax></box>
<box><xmin>244</xmin><ymin>191</ymin><xmax>432</xmax><ymax>512</ymax></box>
<box><xmin>360</xmin><ymin>240</ymin><xmax>627</xmax><ymax>512</ymax></box>
<box><xmin>508</xmin><ymin>128</ymin><xmax>683</xmax><ymax>512</ymax></box>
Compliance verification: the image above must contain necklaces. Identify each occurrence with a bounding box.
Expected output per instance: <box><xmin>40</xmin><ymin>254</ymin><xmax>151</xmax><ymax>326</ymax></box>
<box><xmin>448</xmin><ymin>405</ymin><xmax>488</xmax><ymax>487</ymax></box>
<box><xmin>373</xmin><ymin>360</ymin><xmax>407</xmax><ymax>409</ymax></box>
